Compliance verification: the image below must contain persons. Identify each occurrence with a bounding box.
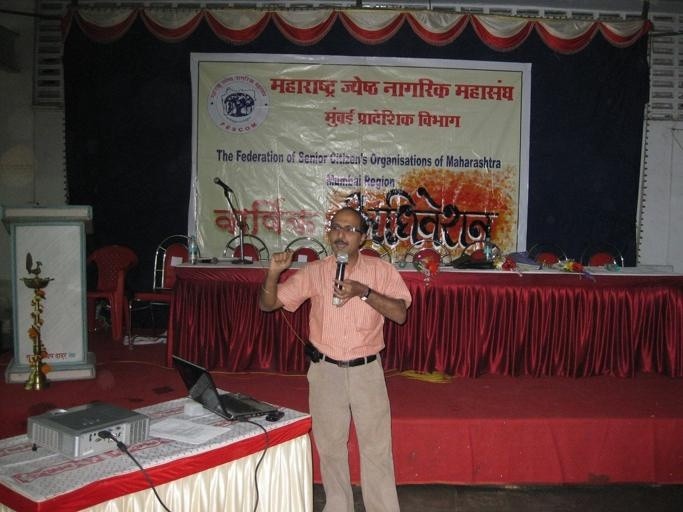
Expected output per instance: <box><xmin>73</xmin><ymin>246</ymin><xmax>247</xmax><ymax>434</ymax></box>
<box><xmin>258</xmin><ymin>208</ymin><xmax>411</xmax><ymax>511</ymax></box>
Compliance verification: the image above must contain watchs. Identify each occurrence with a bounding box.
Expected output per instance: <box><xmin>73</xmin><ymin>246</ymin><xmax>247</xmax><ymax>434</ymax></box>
<box><xmin>359</xmin><ymin>287</ymin><xmax>371</xmax><ymax>301</ymax></box>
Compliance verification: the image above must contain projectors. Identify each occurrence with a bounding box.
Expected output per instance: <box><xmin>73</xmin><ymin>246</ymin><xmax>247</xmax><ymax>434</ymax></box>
<box><xmin>26</xmin><ymin>400</ymin><xmax>151</xmax><ymax>462</ymax></box>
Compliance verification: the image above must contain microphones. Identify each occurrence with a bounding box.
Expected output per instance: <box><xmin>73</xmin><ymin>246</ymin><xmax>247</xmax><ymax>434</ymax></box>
<box><xmin>198</xmin><ymin>257</ymin><xmax>219</xmax><ymax>264</ymax></box>
<box><xmin>332</xmin><ymin>251</ymin><xmax>349</xmax><ymax>308</ymax></box>
<box><xmin>213</xmin><ymin>177</ymin><xmax>235</xmax><ymax>193</ymax></box>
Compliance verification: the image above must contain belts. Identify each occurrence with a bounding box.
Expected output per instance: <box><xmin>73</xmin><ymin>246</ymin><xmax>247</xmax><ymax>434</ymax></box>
<box><xmin>319</xmin><ymin>353</ymin><xmax>376</xmax><ymax>368</ymax></box>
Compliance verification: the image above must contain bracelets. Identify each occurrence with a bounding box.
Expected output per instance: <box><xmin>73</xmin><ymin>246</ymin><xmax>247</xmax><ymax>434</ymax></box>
<box><xmin>260</xmin><ymin>284</ymin><xmax>277</xmax><ymax>294</ymax></box>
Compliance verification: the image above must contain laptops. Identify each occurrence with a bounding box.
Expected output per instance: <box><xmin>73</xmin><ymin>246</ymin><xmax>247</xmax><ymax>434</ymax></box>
<box><xmin>171</xmin><ymin>353</ymin><xmax>278</xmax><ymax>419</ymax></box>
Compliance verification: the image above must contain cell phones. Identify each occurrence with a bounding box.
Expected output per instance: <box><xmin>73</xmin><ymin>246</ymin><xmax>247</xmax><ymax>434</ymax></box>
<box><xmin>267</xmin><ymin>410</ymin><xmax>284</xmax><ymax>421</ymax></box>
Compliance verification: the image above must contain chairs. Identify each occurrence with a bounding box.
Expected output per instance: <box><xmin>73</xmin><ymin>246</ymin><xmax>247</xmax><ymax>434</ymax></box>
<box><xmin>86</xmin><ymin>235</ymin><xmax>202</xmax><ymax>368</ymax></box>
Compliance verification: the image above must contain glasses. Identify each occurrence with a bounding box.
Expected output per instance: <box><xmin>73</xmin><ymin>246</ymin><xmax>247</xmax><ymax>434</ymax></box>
<box><xmin>330</xmin><ymin>224</ymin><xmax>363</xmax><ymax>235</ymax></box>
